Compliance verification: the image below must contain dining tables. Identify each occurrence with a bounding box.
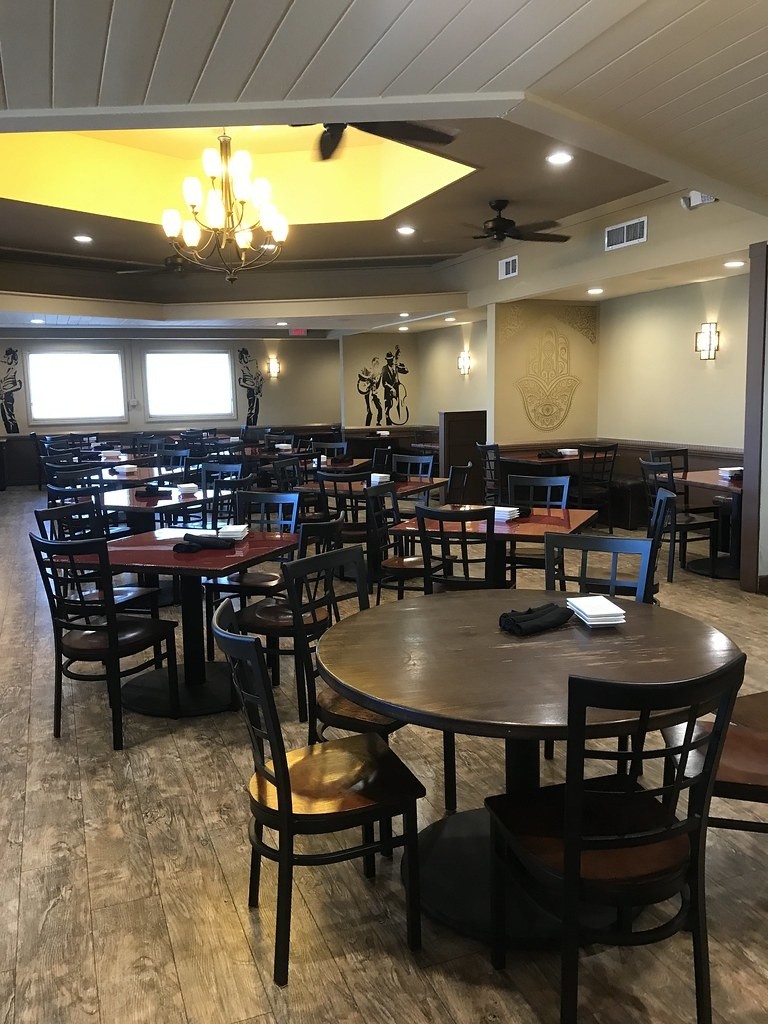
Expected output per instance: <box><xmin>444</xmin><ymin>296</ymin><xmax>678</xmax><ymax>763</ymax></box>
<box><xmin>254</xmin><ymin>456</ymin><xmax>372</xmax><ymax>522</ymax></box>
<box><xmin>292</xmin><ymin>478</ymin><xmax>450</xmax><ymax>580</ymax></box>
<box><xmin>343</xmin><ymin>433</ymin><xmax>423</xmax><ymax>478</ymax></box>
<box><xmin>647</xmin><ymin>469</ymin><xmax>743</xmax><ymax>580</ymax></box>
<box><xmin>386</xmin><ymin>504</ymin><xmax>599</xmax><ymax>589</ymax></box>
<box><xmin>42</xmin><ymin>528</ymin><xmax>320</xmax><ymax>717</ymax></box>
<box><xmin>493</xmin><ymin>449</ymin><xmax>620</xmax><ymax>510</ymax></box>
<box><xmin>70</xmin><ymin>466</ymin><xmax>202</xmax><ymax>528</ymax></box>
<box><xmin>316</xmin><ymin>588</ymin><xmax>743</xmax><ymax>948</ymax></box>
<box><xmin>69</xmin><ymin>431</ymin><xmax>316</xmax><ymax>523</ymax></box>
<box><xmin>409</xmin><ymin>443</ymin><xmax>440</xmax><ymax>499</ymax></box>
<box><xmin>48</xmin><ymin>486</ymin><xmax>243</xmax><ymax>610</ymax></box>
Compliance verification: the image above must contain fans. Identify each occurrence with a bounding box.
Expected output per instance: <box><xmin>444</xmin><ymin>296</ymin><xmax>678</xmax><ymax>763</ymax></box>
<box><xmin>289</xmin><ymin>121</ymin><xmax>456</xmax><ymax>160</ymax></box>
<box><xmin>462</xmin><ymin>199</ymin><xmax>571</xmax><ymax>243</ymax></box>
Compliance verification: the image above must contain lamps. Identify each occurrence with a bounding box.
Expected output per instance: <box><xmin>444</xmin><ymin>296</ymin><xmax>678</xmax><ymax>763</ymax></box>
<box><xmin>695</xmin><ymin>323</ymin><xmax>720</xmax><ymax>360</ymax></box>
<box><xmin>456</xmin><ymin>351</ymin><xmax>470</xmax><ymax>375</ymax></box>
<box><xmin>267</xmin><ymin>355</ymin><xmax>280</xmax><ymax>379</ymax></box>
<box><xmin>162</xmin><ymin>135</ymin><xmax>288</xmax><ymax>282</ymax></box>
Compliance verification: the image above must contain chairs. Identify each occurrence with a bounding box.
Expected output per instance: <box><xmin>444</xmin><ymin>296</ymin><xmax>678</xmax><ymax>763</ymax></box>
<box><xmin>0</xmin><ymin>421</ymin><xmax>768</xmax><ymax>1023</ymax></box>
